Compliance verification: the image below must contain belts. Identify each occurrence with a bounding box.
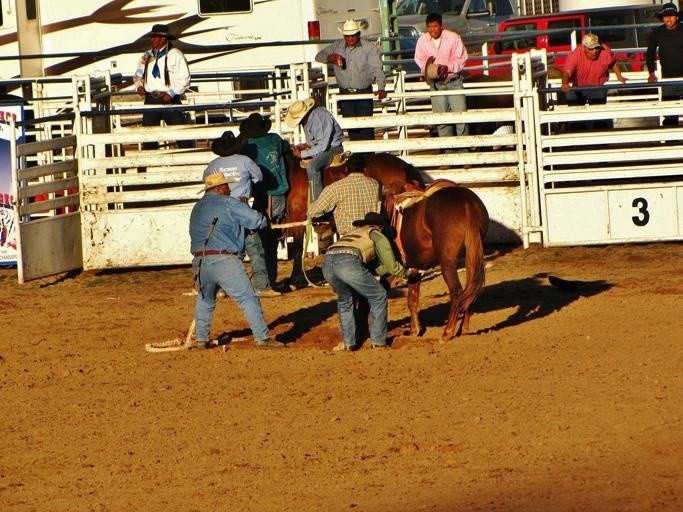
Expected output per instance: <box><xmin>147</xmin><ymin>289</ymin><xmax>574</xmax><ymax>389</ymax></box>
<box><xmin>147</xmin><ymin>92</ymin><xmax>164</xmax><ymax>98</ymax></box>
<box><xmin>431</xmin><ymin>74</ymin><xmax>460</xmax><ymax>85</ymax></box>
<box><xmin>338</xmin><ymin>88</ymin><xmax>370</xmax><ymax>93</ymax></box>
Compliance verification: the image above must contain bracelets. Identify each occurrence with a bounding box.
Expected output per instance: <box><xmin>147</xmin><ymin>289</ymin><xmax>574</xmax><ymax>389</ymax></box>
<box><xmin>168</xmin><ymin>88</ymin><xmax>174</xmax><ymax>96</ymax></box>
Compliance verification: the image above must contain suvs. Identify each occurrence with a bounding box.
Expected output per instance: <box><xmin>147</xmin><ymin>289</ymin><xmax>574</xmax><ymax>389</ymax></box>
<box><xmin>463</xmin><ymin>3</ymin><xmax>683</xmax><ymax>80</ymax></box>
<box><xmin>397</xmin><ymin>0</ymin><xmax>518</xmax><ymax>55</ymax></box>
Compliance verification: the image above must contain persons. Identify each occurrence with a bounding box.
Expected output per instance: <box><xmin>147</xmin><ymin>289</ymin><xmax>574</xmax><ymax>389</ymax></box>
<box><xmin>285</xmin><ymin>99</ymin><xmax>345</xmax><ymax>203</ymax></box>
<box><xmin>413</xmin><ymin>13</ymin><xmax>472</xmax><ymax>169</ymax></box>
<box><xmin>189</xmin><ymin>173</ymin><xmax>284</xmax><ymax>349</ymax></box>
<box><xmin>646</xmin><ymin>3</ymin><xmax>683</xmax><ymax>147</ymax></box>
<box><xmin>240</xmin><ymin>113</ymin><xmax>289</xmax><ymax>283</ymax></box>
<box><xmin>132</xmin><ymin>25</ymin><xmax>196</xmax><ymax>149</ymax></box>
<box><xmin>313</xmin><ymin>20</ymin><xmax>387</xmax><ymax>156</ymax></box>
<box><xmin>309</xmin><ymin>156</ymin><xmax>381</xmax><ymax>237</ymax></box>
<box><xmin>322</xmin><ymin>212</ymin><xmax>417</xmax><ymax>351</ymax></box>
<box><xmin>559</xmin><ymin>33</ymin><xmax>627</xmax><ymax>133</ymax></box>
<box><xmin>202</xmin><ymin>130</ymin><xmax>281</xmax><ymax>297</ymax></box>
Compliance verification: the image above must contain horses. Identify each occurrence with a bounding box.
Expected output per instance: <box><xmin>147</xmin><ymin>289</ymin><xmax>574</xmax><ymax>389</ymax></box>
<box><xmin>251</xmin><ymin>150</ymin><xmax>425</xmax><ymax>293</ymax></box>
<box><xmin>309</xmin><ymin>178</ymin><xmax>490</xmax><ymax>345</ymax></box>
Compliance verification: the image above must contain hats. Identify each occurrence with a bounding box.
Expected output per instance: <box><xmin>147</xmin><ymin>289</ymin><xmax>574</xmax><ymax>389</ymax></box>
<box><xmin>425</xmin><ymin>56</ymin><xmax>441</xmax><ymax>82</ymax></box>
<box><xmin>338</xmin><ymin>19</ymin><xmax>365</xmax><ymax>36</ymax></box>
<box><xmin>197</xmin><ymin>173</ymin><xmax>238</xmax><ymax>195</ymax></box>
<box><xmin>144</xmin><ymin>24</ymin><xmax>177</xmax><ymax>40</ymax></box>
<box><xmin>582</xmin><ymin>32</ymin><xmax>601</xmax><ymax>49</ymax></box>
<box><xmin>656</xmin><ymin>3</ymin><xmax>682</xmax><ymax>23</ymax></box>
<box><xmin>286</xmin><ymin>98</ymin><xmax>314</xmax><ymax>128</ymax></box>
<box><xmin>212</xmin><ymin>131</ymin><xmax>243</xmax><ymax>156</ymax></box>
<box><xmin>240</xmin><ymin>114</ymin><xmax>271</xmax><ymax>138</ymax></box>
<box><xmin>352</xmin><ymin>212</ymin><xmax>397</xmax><ymax>241</ymax></box>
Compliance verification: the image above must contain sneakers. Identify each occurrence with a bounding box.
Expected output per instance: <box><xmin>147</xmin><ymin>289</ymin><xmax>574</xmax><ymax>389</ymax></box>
<box><xmin>257</xmin><ymin>289</ymin><xmax>281</xmax><ymax>296</ymax></box>
<box><xmin>197</xmin><ymin>341</ymin><xmax>211</xmax><ymax>350</ymax></box>
<box><xmin>312</xmin><ymin>214</ymin><xmax>333</xmax><ymax>222</ymax></box>
<box><xmin>217</xmin><ymin>292</ymin><xmax>225</xmax><ymax>298</ymax></box>
<box><xmin>333</xmin><ymin>342</ymin><xmax>353</xmax><ymax>352</ymax></box>
<box><xmin>371</xmin><ymin>345</ymin><xmax>391</xmax><ymax>348</ymax></box>
<box><xmin>255</xmin><ymin>338</ymin><xmax>284</xmax><ymax>347</ymax></box>
